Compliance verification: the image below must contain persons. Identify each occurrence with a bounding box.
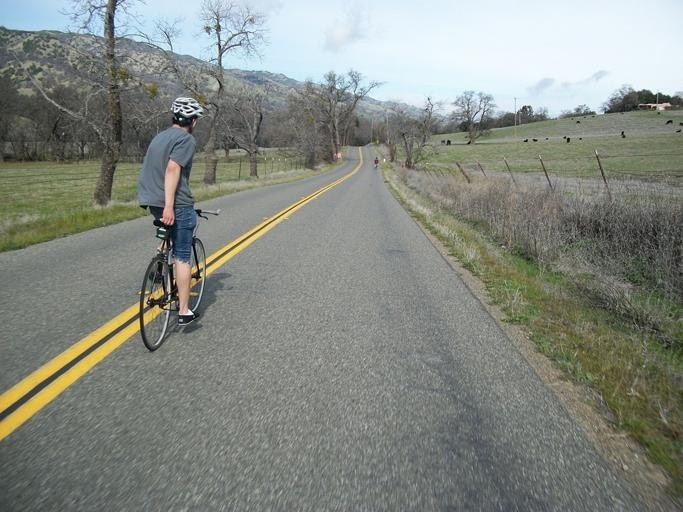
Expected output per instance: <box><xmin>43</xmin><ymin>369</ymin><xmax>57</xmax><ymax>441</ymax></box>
<box><xmin>137</xmin><ymin>96</ymin><xmax>203</xmax><ymax>327</ymax></box>
<box><xmin>373</xmin><ymin>156</ymin><xmax>379</xmax><ymax>169</ymax></box>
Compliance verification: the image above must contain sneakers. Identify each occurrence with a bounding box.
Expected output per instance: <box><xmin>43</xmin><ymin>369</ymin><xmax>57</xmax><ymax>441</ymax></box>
<box><xmin>156</xmin><ymin>247</ymin><xmax>162</xmax><ymax>276</ymax></box>
<box><xmin>177</xmin><ymin>310</ymin><xmax>202</xmax><ymax>326</ymax></box>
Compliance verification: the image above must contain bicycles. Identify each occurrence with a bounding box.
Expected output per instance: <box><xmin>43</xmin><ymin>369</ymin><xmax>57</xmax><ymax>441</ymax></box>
<box><xmin>138</xmin><ymin>207</ymin><xmax>220</xmax><ymax>351</ymax></box>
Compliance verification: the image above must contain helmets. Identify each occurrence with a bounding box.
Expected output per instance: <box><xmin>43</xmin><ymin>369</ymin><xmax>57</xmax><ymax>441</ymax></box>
<box><xmin>171</xmin><ymin>96</ymin><xmax>206</xmax><ymax>120</ymax></box>
